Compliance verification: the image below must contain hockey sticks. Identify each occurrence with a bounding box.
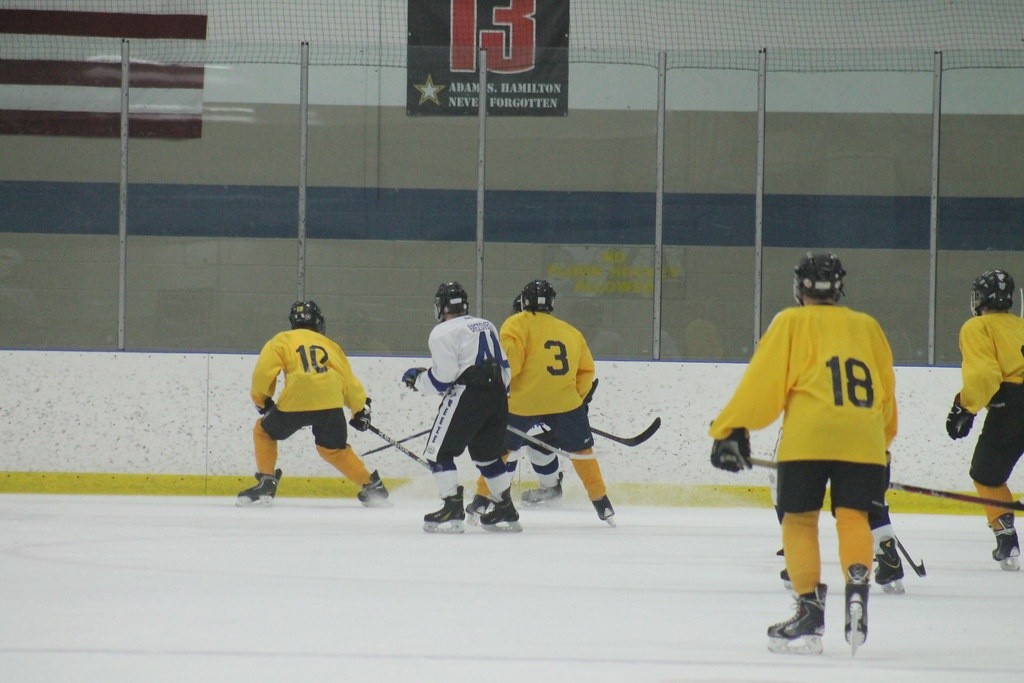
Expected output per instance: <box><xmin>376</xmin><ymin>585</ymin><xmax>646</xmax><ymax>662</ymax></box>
<box><xmin>368</xmin><ymin>423</ymin><xmax>431</xmax><ymax>472</ymax></box>
<box><xmin>358</xmin><ymin>427</ymin><xmax>432</xmax><ymax>458</ymax></box>
<box><xmin>894</xmin><ymin>535</ymin><xmax>927</xmax><ymax>579</ymax></box>
<box><xmin>505</xmin><ymin>423</ymin><xmax>608</xmax><ymax>460</ymax></box>
<box><xmin>719</xmin><ymin>453</ymin><xmax>1024</xmax><ymax>513</ymax></box>
<box><xmin>589</xmin><ymin>416</ymin><xmax>662</xmax><ymax>447</ymax></box>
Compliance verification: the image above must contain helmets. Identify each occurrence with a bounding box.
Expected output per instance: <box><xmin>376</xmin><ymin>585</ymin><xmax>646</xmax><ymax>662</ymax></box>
<box><xmin>513</xmin><ymin>279</ymin><xmax>557</xmax><ymax>313</ymax></box>
<box><xmin>971</xmin><ymin>269</ymin><xmax>1015</xmax><ymax>314</ymax></box>
<box><xmin>792</xmin><ymin>251</ymin><xmax>847</xmax><ymax>306</ymax></box>
<box><xmin>434</xmin><ymin>280</ymin><xmax>470</xmax><ymax>319</ymax></box>
<box><xmin>289</xmin><ymin>301</ymin><xmax>325</xmax><ymax>335</ymax></box>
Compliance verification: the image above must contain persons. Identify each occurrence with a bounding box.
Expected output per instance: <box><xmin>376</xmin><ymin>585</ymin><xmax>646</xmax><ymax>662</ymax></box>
<box><xmin>465</xmin><ymin>281</ymin><xmax>616</xmax><ymax>531</ymax></box>
<box><xmin>710</xmin><ymin>251</ymin><xmax>904</xmax><ymax>654</ymax></box>
<box><xmin>401</xmin><ymin>282</ymin><xmax>521</xmax><ymax>533</ymax></box>
<box><xmin>234</xmin><ymin>301</ymin><xmax>394</xmax><ymax>510</ymax></box>
<box><xmin>945</xmin><ymin>267</ymin><xmax>1023</xmax><ymax>571</ymax></box>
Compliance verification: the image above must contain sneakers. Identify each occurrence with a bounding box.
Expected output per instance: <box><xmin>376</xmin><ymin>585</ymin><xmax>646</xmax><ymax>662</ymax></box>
<box><xmin>992</xmin><ymin>512</ymin><xmax>1023</xmax><ymax>571</ymax></box>
<box><xmin>768</xmin><ymin>581</ymin><xmax>830</xmax><ymax>655</ymax></box>
<box><xmin>358</xmin><ymin>469</ymin><xmax>395</xmax><ymax>508</ymax></box>
<box><xmin>846</xmin><ymin>563</ymin><xmax>867</xmax><ymax>657</ymax></box>
<box><xmin>592</xmin><ymin>495</ymin><xmax>620</xmax><ymax>527</ymax></box>
<box><xmin>480</xmin><ymin>489</ymin><xmax>524</xmax><ymax>534</ymax></box>
<box><xmin>235</xmin><ymin>469</ymin><xmax>280</xmax><ymax>507</ymax></box>
<box><xmin>873</xmin><ymin>536</ymin><xmax>910</xmax><ymax>593</ymax></box>
<box><xmin>424</xmin><ymin>486</ymin><xmax>465</xmax><ymax>534</ymax></box>
<box><xmin>776</xmin><ymin>548</ymin><xmax>789</xmax><ymax>586</ymax></box>
<box><xmin>521</xmin><ymin>472</ymin><xmax>565</xmax><ymax>510</ymax></box>
<box><xmin>467</xmin><ymin>495</ymin><xmax>490</xmax><ymax>517</ymax></box>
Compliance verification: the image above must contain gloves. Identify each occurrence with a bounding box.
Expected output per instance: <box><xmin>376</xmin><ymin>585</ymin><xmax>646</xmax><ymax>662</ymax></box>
<box><xmin>402</xmin><ymin>368</ymin><xmax>424</xmax><ymax>391</ymax></box>
<box><xmin>257</xmin><ymin>400</ymin><xmax>275</xmax><ymax>415</ymax></box>
<box><xmin>945</xmin><ymin>394</ymin><xmax>976</xmax><ymax>439</ymax></box>
<box><xmin>711</xmin><ymin>431</ymin><xmax>752</xmax><ymax>471</ymax></box>
<box><xmin>351</xmin><ymin>404</ymin><xmax>372</xmax><ymax>431</ymax></box>
